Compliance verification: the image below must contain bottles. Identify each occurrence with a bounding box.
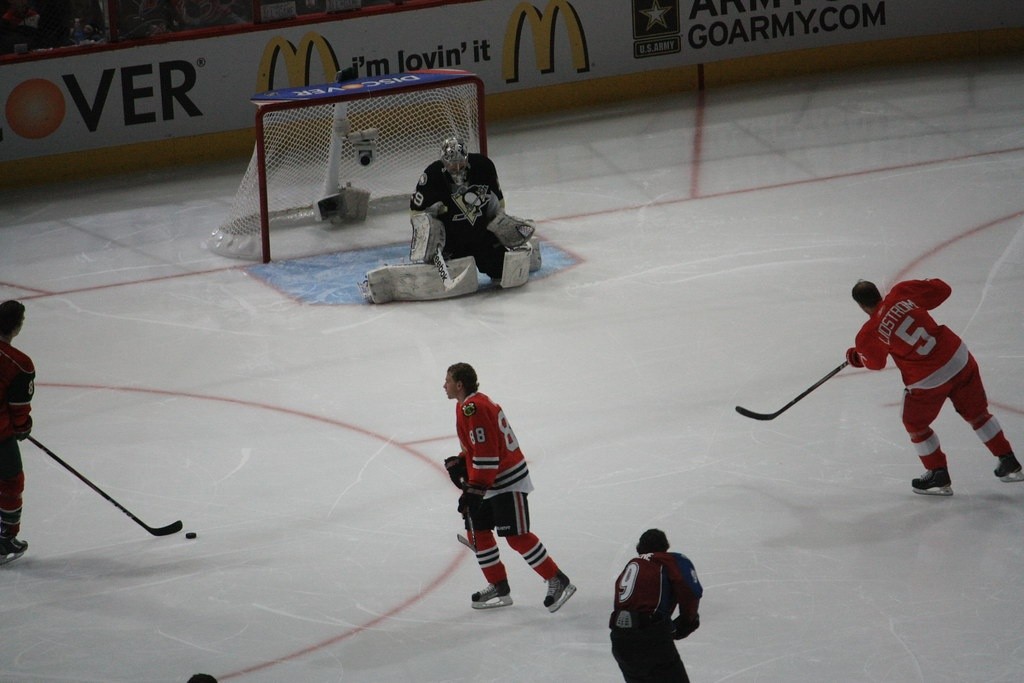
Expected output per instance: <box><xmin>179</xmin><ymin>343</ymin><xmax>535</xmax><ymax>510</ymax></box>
<box><xmin>74</xmin><ymin>18</ymin><xmax>83</xmax><ymax>45</ymax></box>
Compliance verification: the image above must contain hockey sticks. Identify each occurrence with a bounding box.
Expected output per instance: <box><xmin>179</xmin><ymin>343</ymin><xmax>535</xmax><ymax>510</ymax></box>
<box><xmin>432</xmin><ymin>247</ymin><xmax>472</xmax><ymax>291</ymax></box>
<box><xmin>26</xmin><ymin>434</ymin><xmax>184</xmax><ymax>538</ymax></box>
<box><xmin>457</xmin><ymin>476</ymin><xmax>479</xmax><ymax>554</ymax></box>
<box><xmin>735</xmin><ymin>361</ymin><xmax>849</xmax><ymax>422</ymax></box>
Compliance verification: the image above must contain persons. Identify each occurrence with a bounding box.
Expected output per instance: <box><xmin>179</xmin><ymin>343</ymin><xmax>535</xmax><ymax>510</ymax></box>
<box><xmin>0</xmin><ymin>300</ymin><xmax>35</xmax><ymax>569</ymax></box>
<box><xmin>410</xmin><ymin>136</ymin><xmax>535</xmax><ymax>290</ymax></box>
<box><xmin>609</xmin><ymin>528</ymin><xmax>703</xmax><ymax>683</ymax></box>
<box><xmin>0</xmin><ymin>0</ymin><xmax>72</xmax><ymax>53</ymax></box>
<box><xmin>187</xmin><ymin>672</ymin><xmax>219</xmax><ymax>683</ymax></box>
<box><xmin>443</xmin><ymin>362</ymin><xmax>571</xmax><ymax>607</ymax></box>
<box><xmin>845</xmin><ymin>278</ymin><xmax>1023</xmax><ymax>491</ymax></box>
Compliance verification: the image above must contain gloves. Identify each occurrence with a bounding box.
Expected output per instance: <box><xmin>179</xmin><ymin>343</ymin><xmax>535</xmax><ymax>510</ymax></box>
<box><xmin>457</xmin><ymin>489</ymin><xmax>483</xmax><ymax>520</ymax></box>
<box><xmin>670</xmin><ymin>611</ymin><xmax>699</xmax><ymax>640</ymax></box>
<box><xmin>444</xmin><ymin>456</ymin><xmax>469</xmax><ymax>491</ymax></box>
<box><xmin>846</xmin><ymin>347</ymin><xmax>864</xmax><ymax>369</ymax></box>
<box><xmin>12</xmin><ymin>416</ymin><xmax>32</xmax><ymax>440</ymax></box>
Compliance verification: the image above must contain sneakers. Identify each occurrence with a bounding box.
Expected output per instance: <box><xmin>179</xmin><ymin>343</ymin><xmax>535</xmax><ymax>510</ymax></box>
<box><xmin>473</xmin><ymin>580</ymin><xmax>513</xmax><ymax>609</ymax></box>
<box><xmin>543</xmin><ymin>569</ymin><xmax>577</xmax><ymax>612</ymax></box>
<box><xmin>0</xmin><ymin>536</ymin><xmax>27</xmax><ymax>565</ymax></box>
<box><xmin>911</xmin><ymin>466</ymin><xmax>953</xmax><ymax>496</ymax></box>
<box><xmin>993</xmin><ymin>452</ymin><xmax>1024</xmax><ymax>482</ymax></box>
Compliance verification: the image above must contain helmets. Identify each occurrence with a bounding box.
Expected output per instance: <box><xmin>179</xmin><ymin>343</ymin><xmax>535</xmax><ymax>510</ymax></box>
<box><xmin>440</xmin><ymin>138</ymin><xmax>469</xmax><ymax>186</ymax></box>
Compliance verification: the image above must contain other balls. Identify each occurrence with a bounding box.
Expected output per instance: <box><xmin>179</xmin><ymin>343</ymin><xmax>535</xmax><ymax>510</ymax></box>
<box><xmin>186</xmin><ymin>532</ymin><xmax>197</xmax><ymax>539</ymax></box>
